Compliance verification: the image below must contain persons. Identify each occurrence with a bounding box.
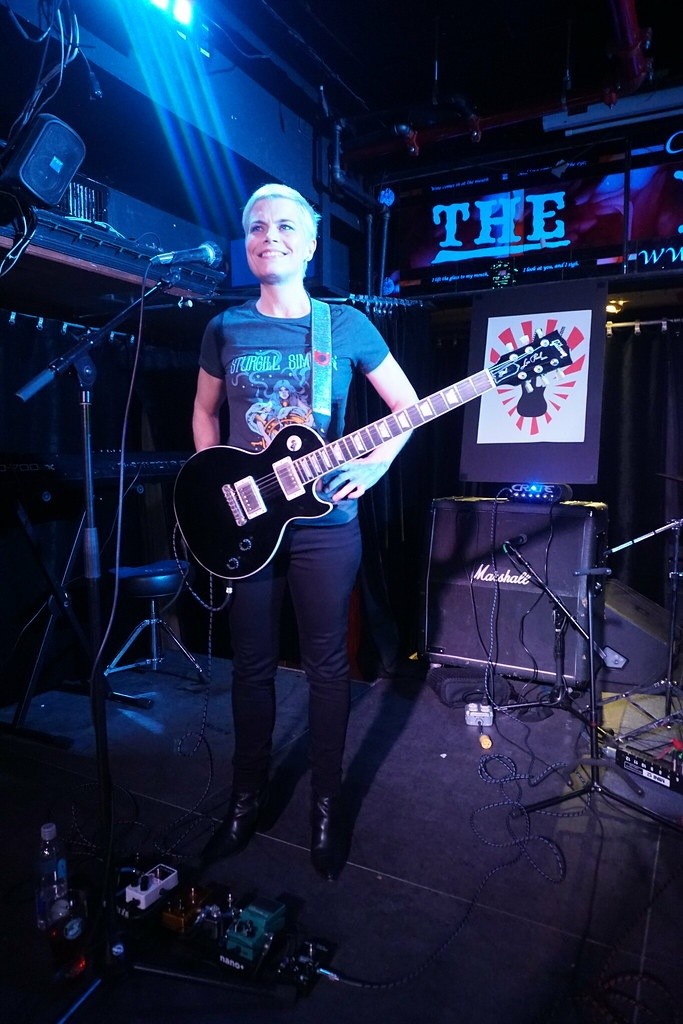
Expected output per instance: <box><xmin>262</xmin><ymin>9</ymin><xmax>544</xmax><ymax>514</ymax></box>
<box><xmin>192</xmin><ymin>182</ymin><xmax>421</xmax><ymax>882</ymax></box>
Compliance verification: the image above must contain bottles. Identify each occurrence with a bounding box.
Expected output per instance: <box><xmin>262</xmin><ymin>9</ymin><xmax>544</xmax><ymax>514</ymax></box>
<box><xmin>36</xmin><ymin>823</ymin><xmax>72</xmax><ymax>929</ymax></box>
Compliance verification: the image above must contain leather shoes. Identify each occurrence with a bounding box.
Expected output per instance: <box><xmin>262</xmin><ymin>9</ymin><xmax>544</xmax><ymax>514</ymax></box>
<box><xmin>311</xmin><ymin>793</ymin><xmax>347</xmax><ymax>881</ymax></box>
<box><xmin>199</xmin><ymin>783</ymin><xmax>271</xmax><ymax>861</ymax></box>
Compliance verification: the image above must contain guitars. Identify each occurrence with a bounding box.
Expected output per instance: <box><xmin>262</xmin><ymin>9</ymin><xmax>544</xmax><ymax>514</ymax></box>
<box><xmin>172</xmin><ymin>327</ymin><xmax>572</xmax><ymax>580</ymax></box>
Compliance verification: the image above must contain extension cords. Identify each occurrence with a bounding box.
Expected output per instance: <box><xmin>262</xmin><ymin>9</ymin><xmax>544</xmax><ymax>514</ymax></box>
<box><xmin>465</xmin><ymin>702</ymin><xmax>493</xmax><ymax>727</ymax></box>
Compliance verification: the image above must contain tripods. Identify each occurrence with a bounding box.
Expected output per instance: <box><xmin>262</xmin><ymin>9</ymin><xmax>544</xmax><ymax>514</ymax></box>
<box><xmin>492</xmin><ymin>518</ymin><xmax>683</xmax><ymax>836</ymax></box>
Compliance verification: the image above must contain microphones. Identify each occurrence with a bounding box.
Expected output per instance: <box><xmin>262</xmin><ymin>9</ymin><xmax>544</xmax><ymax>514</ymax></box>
<box><xmin>150</xmin><ymin>240</ymin><xmax>223</xmax><ymax>268</ymax></box>
<box><xmin>504</xmin><ymin>534</ymin><xmax>527</xmax><ymax>545</ymax></box>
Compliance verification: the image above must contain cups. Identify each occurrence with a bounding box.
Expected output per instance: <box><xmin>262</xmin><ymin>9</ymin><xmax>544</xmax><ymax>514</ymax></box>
<box><xmin>48</xmin><ymin>887</ymin><xmax>101</xmax><ymax>975</ymax></box>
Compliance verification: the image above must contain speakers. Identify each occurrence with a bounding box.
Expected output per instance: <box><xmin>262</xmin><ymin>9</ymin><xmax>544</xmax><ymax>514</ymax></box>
<box><xmin>0</xmin><ymin>112</ymin><xmax>86</xmax><ymax>208</ymax></box>
<box><xmin>416</xmin><ymin>495</ymin><xmax>609</xmax><ymax>689</ymax></box>
